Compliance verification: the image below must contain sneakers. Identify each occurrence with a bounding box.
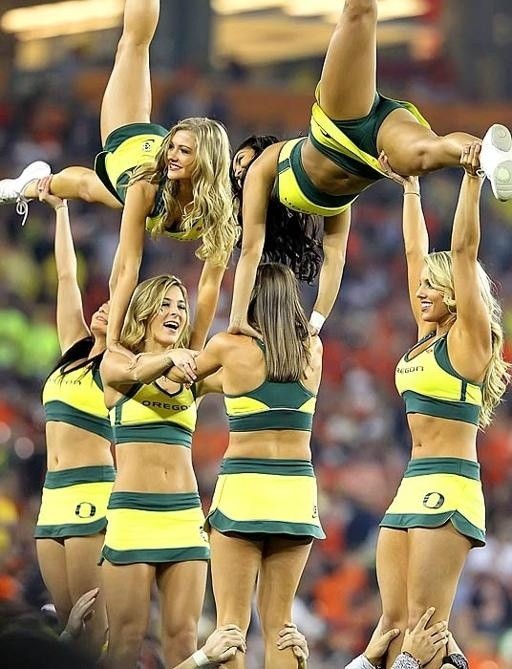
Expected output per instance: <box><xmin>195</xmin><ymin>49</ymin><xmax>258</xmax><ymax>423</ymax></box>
<box><xmin>0</xmin><ymin>159</ymin><xmax>53</xmax><ymax>226</ymax></box>
<box><xmin>478</xmin><ymin>124</ymin><xmax>512</xmax><ymax>202</ymax></box>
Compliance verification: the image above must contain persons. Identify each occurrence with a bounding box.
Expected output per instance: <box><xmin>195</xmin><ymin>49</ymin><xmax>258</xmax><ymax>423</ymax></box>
<box><xmin>1</xmin><ymin>1</ymin><xmax>512</xmax><ymax>669</ymax></box>
